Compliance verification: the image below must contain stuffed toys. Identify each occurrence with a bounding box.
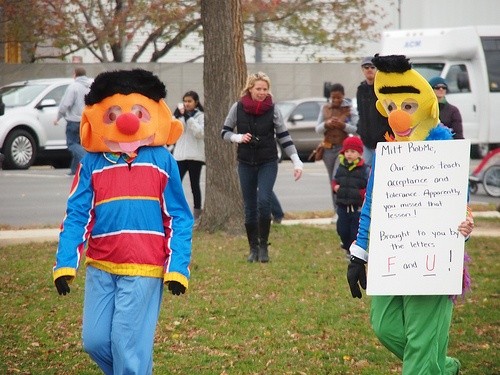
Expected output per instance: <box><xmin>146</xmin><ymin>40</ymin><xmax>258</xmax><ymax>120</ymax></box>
<box><xmin>51</xmin><ymin>68</ymin><xmax>194</xmax><ymax>375</ymax></box>
<box><xmin>346</xmin><ymin>46</ymin><xmax>475</xmax><ymax>374</ymax></box>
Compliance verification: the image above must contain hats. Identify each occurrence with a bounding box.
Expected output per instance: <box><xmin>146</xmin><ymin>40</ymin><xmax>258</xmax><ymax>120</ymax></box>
<box><xmin>339</xmin><ymin>136</ymin><xmax>363</xmax><ymax>155</ymax></box>
<box><xmin>361</xmin><ymin>56</ymin><xmax>374</xmax><ymax>66</ymax></box>
<box><xmin>429</xmin><ymin>77</ymin><xmax>448</xmax><ymax>87</ymax></box>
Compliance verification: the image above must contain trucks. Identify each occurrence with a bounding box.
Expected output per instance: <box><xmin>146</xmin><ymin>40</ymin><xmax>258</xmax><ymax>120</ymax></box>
<box><xmin>378</xmin><ymin>24</ymin><xmax>500</xmax><ymax>160</ymax></box>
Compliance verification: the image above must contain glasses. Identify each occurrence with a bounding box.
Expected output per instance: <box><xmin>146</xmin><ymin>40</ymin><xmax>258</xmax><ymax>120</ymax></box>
<box><xmin>365</xmin><ymin>66</ymin><xmax>375</xmax><ymax>69</ymax></box>
<box><xmin>433</xmin><ymin>86</ymin><xmax>448</xmax><ymax>90</ymax></box>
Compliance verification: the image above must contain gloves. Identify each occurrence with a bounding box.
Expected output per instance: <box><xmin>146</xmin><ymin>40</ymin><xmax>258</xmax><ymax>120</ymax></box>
<box><xmin>168</xmin><ymin>281</ymin><xmax>186</xmax><ymax>296</ymax></box>
<box><xmin>346</xmin><ymin>256</ymin><xmax>367</xmax><ymax>299</ymax></box>
<box><xmin>56</xmin><ymin>276</ymin><xmax>71</xmax><ymax>296</ymax></box>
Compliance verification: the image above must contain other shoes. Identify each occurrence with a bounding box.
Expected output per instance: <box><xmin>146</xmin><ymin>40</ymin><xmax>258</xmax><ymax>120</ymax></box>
<box><xmin>194</xmin><ymin>209</ymin><xmax>202</xmax><ymax>220</ymax></box>
<box><xmin>66</xmin><ymin>173</ymin><xmax>75</xmax><ymax>177</ymax></box>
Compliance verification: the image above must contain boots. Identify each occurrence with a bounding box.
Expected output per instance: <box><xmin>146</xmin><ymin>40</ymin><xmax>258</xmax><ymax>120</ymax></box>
<box><xmin>258</xmin><ymin>218</ymin><xmax>272</xmax><ymax>263</ymax></box>
<box><xmin>244</xmin><ymin>223</ymin><xmax>258</xmax><ymax>262</ymax></box>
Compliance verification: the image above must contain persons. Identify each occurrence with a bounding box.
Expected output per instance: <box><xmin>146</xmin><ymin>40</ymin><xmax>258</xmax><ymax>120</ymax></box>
<box><xmin>218</xmin><ymin>72</ymin><xmax>303</xmax><ymax>263</ymax></box>
<box><xmin>426</xmin><ymin>75</ymin><xmax>465</xmax><ymax>144</ymax></box>
<box><xmin>266</xmin><ymin>194</ymin><xmax>285</xmax><ymax>225</ymax></box>
<box><xmin>332</xmin><ymin>137</ymin><xmax>371</xmax><ymax>250</ymax></box>
<box><xmin>354</xmin><ymin>56</ymin><xmax>397</xmax><ymax>173</ymax></box>
<box><xmin>54</xmin><ymin>68</ymin><xmax>96</xmax><ymax>175</ymax></box>
<box><xmin>167</xmin><ymin>91</ymin><xmax>209</xmax><ymax>218</ymax></box>
<box><xmin>316</xmin><ymin>81</ymin><xmax>360</xmax><ymax>225</ymax></box>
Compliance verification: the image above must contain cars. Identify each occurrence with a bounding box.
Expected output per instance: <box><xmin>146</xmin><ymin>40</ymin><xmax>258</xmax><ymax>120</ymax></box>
<box><xmin>0</xmin><ymin>78</ymin><xmax>92</xmax><ymax>170</ymax></box>
<box><xmin>274</xmin><ymin>95</ymin><xmax>353</xmax><ymax>158</ymax></box>
